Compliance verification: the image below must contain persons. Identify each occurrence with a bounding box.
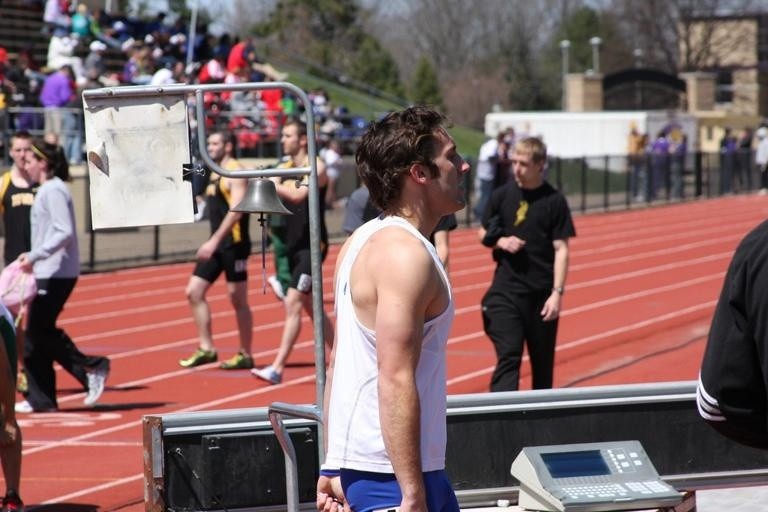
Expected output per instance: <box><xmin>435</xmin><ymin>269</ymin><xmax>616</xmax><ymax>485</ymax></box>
<box><xmin>695</xmin><ymin>219</ymin><xmax>768</xmax><ymax>452</ymax></box>
<box><xmin>624</xmin><ymin>119</ymin><xmax>768</xmax><ymax>204</ymax></box>
<box><xmin>313</xmin><ymin>107</ymin><xmax>471</xmax><ymax>512</ymax></box>
<box><xmin>2</xmin><ymin>0</ymin><xmax>521</xmax><ymax>232</ymax></box>
<box><xmin>479</xmin><ymin>136</ymin><xmax>576</xmax><ymax>391</ymax></box>
<box><xmin>1</xmin><ymin>129</ymin><xmax>110</xmax><ymax>510</ymax></box>
<box><xmin>180</xmin><ymin>122</ymin><xmax>334</xmax><ymax>384</ymax></box>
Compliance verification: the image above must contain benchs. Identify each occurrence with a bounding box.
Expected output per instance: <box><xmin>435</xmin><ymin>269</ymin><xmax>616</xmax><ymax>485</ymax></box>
<box><xmin>0</xmin><ymin>0</ymin><xmax>128</xmax><ymax>85</ymax></box>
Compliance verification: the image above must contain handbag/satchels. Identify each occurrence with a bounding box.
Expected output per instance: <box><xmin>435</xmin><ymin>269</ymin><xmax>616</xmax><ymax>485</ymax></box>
<box><xmin>0</xmin><ymin>259</ymin><xmax>37</xmax><ymax>308</ymax></box>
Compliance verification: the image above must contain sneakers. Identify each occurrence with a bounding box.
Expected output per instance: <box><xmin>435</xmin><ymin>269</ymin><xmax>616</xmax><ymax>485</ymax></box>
<box><xmin>2</xmin><ymin>491</ymin><xmax>24</xmax><ymax>512</ymax></box>
<box><xmin>268</xmin><ymin>275</ymin><xmax>285</xmax><ymax>300</ymax></box>
<box><xmin>84</xmin><ymin>357</ymin><xmax>108</xmax><ymax>405</ymax></box>
<box><xmin>220</xmin><ymin>352</ymin><xmax>253</xmax><ymax>370</ymax></box>
<box><xmin>252</xmin><ymin>368</ymin><xmax>280</xmax><ymax>384</ymax></box>
<box><xmin>179</xmin><ymin>350</ymin><xmax>218</xmax><ymax>367</ymax></box>
<box><xmin>15</xmin><ymin>372</ymin><xmax>59</xmax><ymax>412</ymax></box>
